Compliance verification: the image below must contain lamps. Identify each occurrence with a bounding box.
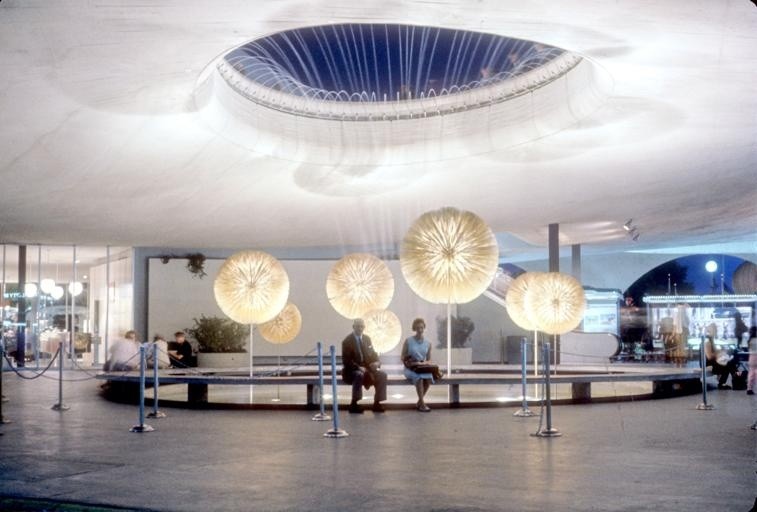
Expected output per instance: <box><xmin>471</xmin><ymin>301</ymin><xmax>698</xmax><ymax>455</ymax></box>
<box><xmin>624</xmin><ymin>219</ymin><xmax>640</xmax><ymax>242</ymax></box>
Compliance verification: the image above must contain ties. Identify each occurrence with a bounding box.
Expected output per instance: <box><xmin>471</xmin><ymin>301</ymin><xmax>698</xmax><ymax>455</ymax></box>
<box><xmin>358</xmin><ymin>336</ymin><xmax>367</xmax><ymax>364</ymax></box>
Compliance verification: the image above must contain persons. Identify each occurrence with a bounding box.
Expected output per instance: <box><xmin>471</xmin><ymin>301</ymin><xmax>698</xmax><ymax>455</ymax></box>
<box><xmin>1</xmin><ymin>324</ymin><xmax>193</xmax><ymax>369</ymax></box>
<box><xmin>620</xmin><ymin>294</ymin><xmax>757</xmax><ymax>396</ymax></box>
<box><xmin>744</xmin><ymin>324</ymin><xmax>757</xmax><ymax>432</ymax></box>
<box><xmin>400</xmin><ymin>317</ymin><xmax>436</xmax><ymax>412</ymax></box>
<box><xmin>340</xmin><ymin>316</ymin><xmax>386</xmax><ymax>414</ymax></box>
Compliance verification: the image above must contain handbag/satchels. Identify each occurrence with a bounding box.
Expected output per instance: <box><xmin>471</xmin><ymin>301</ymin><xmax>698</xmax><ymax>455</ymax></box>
<box><xmin>410</xmin><ymin>365</ymin><xmax>439</xmax><ymax>373</ymax></box>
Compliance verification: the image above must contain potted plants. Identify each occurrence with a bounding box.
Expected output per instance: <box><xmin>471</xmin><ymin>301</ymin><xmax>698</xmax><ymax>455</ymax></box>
<box><xmin>185</xmin><ymin>314</ymin><xmax>257</xmax><ymax>368</ymax></box>
<box><xmin>435</xmin><ymin>315</ymin><xmax>475</xmax><ymax>365</ymax></box>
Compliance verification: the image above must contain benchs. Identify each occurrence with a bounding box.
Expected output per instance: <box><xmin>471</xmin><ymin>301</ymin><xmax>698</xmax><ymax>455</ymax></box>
<box><xmin>95</xmin><ymin>356</ymin><xmax>703</xmax><ymax>409</ymax></box>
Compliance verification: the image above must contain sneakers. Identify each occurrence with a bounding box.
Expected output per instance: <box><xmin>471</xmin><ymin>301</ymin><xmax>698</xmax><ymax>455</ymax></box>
<box><xmin>416</xmin><ymin>402</ymin><xmax>431</xmax><ymax>411</ymax></box>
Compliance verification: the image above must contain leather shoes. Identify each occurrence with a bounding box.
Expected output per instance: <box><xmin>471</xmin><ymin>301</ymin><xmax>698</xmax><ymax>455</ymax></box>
<box><xmin>348</xmin><ymin>404</ymin><xmax>385</xmax><ymax>412</ymax></box>
